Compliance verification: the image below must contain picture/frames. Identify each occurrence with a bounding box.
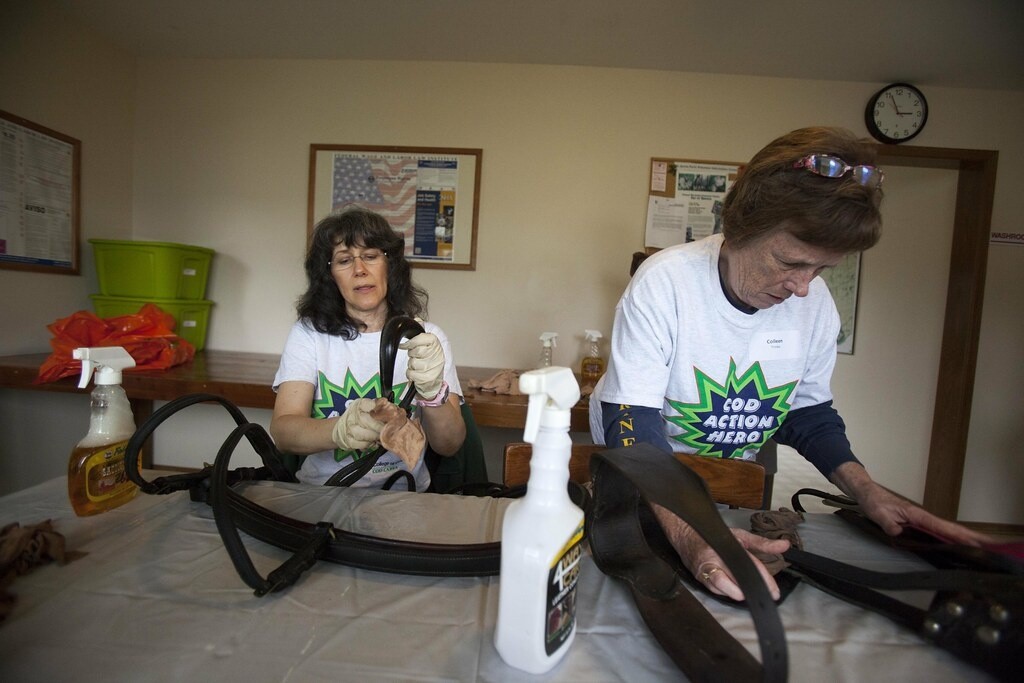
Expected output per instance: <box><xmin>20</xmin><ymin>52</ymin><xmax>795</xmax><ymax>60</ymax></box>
<box><xmin>308</xmin><ymin>144</ymin><xmax>484</xmax><ymax>271</ymax></box>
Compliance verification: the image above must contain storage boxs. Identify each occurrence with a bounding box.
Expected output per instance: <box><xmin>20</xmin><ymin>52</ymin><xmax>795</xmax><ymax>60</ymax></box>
<box><xmin>89</xmin><ymin>295</ymin><xmax>213</xmax><ymax>351</ymax></box>
<box><xmin>87</xmin><ymin>239</ymin><xmax>215</xmax><ymax>300</ymax></box>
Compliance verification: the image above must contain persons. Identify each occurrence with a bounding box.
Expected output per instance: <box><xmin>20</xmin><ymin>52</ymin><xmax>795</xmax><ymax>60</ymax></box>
<box><xmin>587</xmin><ymin>127</ymin><xmax>990</xmax><ymax>605</ymax></box>
<box><xmin>270</xmin><ymin>210</ymin><xmax>465</xmax><ymax>494</ymax></box>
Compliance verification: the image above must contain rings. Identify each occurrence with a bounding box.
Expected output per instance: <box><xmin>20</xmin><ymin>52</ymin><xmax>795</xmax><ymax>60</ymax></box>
<box><xmin>702</xmin><ymin>567</ymin><xmax>718</xmax><ymax>579</ymax></box>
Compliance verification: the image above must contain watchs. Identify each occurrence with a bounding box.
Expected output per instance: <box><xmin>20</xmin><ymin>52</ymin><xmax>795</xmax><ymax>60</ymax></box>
<box><xmin>417</xmin><ymin>381</ymin><xmax>449</xmax><ymax>406</ymax></box>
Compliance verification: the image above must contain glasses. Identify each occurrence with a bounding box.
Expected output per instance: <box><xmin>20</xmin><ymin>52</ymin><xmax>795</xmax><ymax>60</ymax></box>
<box><xmin>794</xmin><ymin>152</ymin><xmax>885</xmax><ymax>190</ymax></box>
<box><xmin>327</xmin><ymin>251</ymin><xmax>387</xmax><ymax>270</ymax></box>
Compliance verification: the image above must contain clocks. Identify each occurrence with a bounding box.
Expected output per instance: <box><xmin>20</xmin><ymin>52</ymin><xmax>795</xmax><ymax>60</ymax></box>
<box><xmin>866</xmin><ymin>82</ymin><xmax>928</xmax><ymax>144</ymax></box>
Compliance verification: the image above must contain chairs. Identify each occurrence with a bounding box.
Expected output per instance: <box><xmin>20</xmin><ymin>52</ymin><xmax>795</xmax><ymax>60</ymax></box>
<box><xmin>502</xmin><ymin>442</ymin><xmax>765</xmax><ymax>510</ymax></box>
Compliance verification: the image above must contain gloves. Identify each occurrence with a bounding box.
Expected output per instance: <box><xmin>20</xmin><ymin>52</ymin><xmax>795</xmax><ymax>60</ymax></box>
<box><xmin>399</xmin><ymin>317</ymin><xmax>445</xmax><ymax>399</ymax></box>
<box><xmin>332</xmin><ymin>398</ymin><xmax>386</xmax><ymax>450</ymax></box>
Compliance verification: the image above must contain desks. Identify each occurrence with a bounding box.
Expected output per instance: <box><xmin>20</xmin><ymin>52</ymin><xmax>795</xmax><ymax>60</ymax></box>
<box><xmin>0</xmin><ymin>350</ymin><xmax>591</xmax><ymax>466</ymax></box>
<box><xmin>0</xmin><ymin>468</ymin><xmax>1024</xmax><ymax>683</ymax></box>
